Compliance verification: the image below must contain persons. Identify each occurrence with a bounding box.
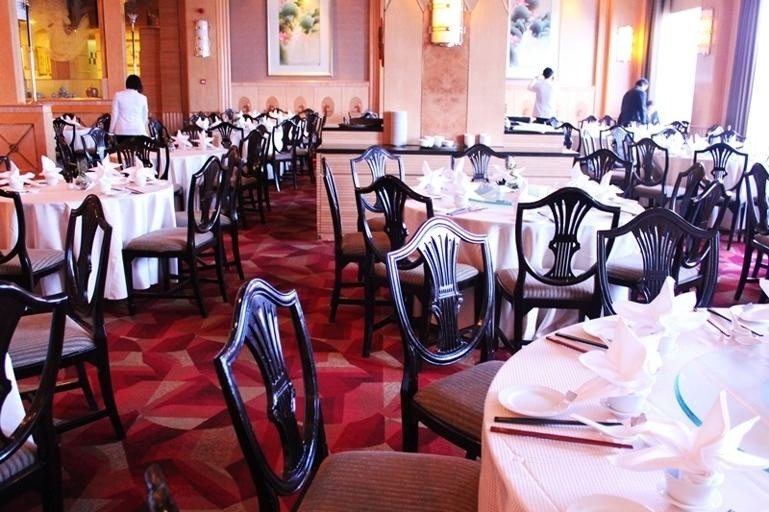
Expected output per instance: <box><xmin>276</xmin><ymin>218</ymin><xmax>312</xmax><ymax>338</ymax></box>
<box><xmin>526</xmin><ymin>66</ymin><xmax>560</xmax><ymax>122</ymax></box>
<box><xmin>617</xmin><ymin>77</ymin><xmax>652</xmax><ymax>128</ymax></box>
<box><xmin>104</xmin><ymin>72</ymin><xmax>152</xmax><ymax>154</ymax></box>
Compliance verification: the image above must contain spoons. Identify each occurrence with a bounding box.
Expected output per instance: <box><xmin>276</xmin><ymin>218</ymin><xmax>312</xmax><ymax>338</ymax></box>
<box><xmin>568</xmin><ymin>408</ymin><xmax>641</xmax><ymax>442</ymax></box>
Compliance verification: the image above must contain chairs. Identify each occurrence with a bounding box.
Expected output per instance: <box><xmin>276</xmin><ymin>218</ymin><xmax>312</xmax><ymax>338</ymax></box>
<box><xmin>2</xmin><ymin>108</ymin><xmax>329</xmax><ymax>512</ymax></box>
<box><xmin>212</xmin><ymin>110</ymin><xmax>769</xmax><ymax>511</ymax></box>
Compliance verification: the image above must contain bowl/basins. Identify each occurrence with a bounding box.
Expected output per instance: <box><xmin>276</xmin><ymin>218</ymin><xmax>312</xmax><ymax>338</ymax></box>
<box><xmin>350</xmin><ymin>118</ymin><xmax>385</xmax><ymax>127</ymax></box>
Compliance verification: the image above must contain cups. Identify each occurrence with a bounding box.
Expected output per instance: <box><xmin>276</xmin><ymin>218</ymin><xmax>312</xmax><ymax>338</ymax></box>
<box><xmin>479</xmin><ymin>132</ymin><xmax>492</xmax><ymax>148</ymax></box>
<box><xmin>463</xmin><ymin>132</ymin><xmax>475</xmax><ymax>148</ymax></box>
<box><xmin>0</xmin><ymin>160</ymin><xmax>157</xmax><ymax>196</ymax></box>
<box><xmin>664</xmin><ymin>461</ymin><xmax>721</xmax><ymax>503</ymax></box>
<box><xmin>410</xmin><ymin>174</ymin><xmax>642</xmax><ymax>224</ymax></box>
<box><xmin>419</xmin><ymin>136</ymin><xmax>455</xmax><ymax>148</ymax></box>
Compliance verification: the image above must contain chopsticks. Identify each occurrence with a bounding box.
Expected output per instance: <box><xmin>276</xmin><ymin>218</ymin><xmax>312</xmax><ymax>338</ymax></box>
<box><xmin>698</xmin><ymin>307</ymin><xmax>765</xmax><ymax>349</ymax></box>
<box><xmin>484</xmin><ymin>410</ymin><xmax>632</xmax><ymax>451</ymax></box>
<box><xmin>545</xmin><ymin>325</ymin><xmax>615</xmax><ymax>357</ymax></box>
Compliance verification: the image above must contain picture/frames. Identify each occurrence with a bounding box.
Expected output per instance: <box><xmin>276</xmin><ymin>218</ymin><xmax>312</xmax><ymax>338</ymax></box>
<box><xmin>266</xmin><ymin>0</ymin><xmax>334</xmax><ymax>76</ymax></box>
<box><xmin>506</xmin><ymin>0</ymin><xmax>563</xmax><ymax>80</ymax></box>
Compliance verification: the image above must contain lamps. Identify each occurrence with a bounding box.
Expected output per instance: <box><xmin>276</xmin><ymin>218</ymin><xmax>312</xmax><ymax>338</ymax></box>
<box><xmin>194</xmin><ymin>20</ymin><xmax>210</xmax><ymax>58</ymax></box>
<box><xmin>431</xmin><ymin>0</ymin><xmax>463</xmax><ymax>47</ymax></box>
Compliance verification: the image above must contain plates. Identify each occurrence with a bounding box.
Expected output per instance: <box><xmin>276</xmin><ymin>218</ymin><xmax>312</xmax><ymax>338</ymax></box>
<box><xmin>730</xmin><ymin>302</ymin><xmax>769</xmax><ymax>321</ymax></box>
<box><xmin>496</xmin><ymin>373</ymin><xmax>572</xmax><ymax>422</ymax></box>
<box><xmin>653</xmin><ymin>475</ymin><xmax>741</xmax><ymax>512</ymax></box>
